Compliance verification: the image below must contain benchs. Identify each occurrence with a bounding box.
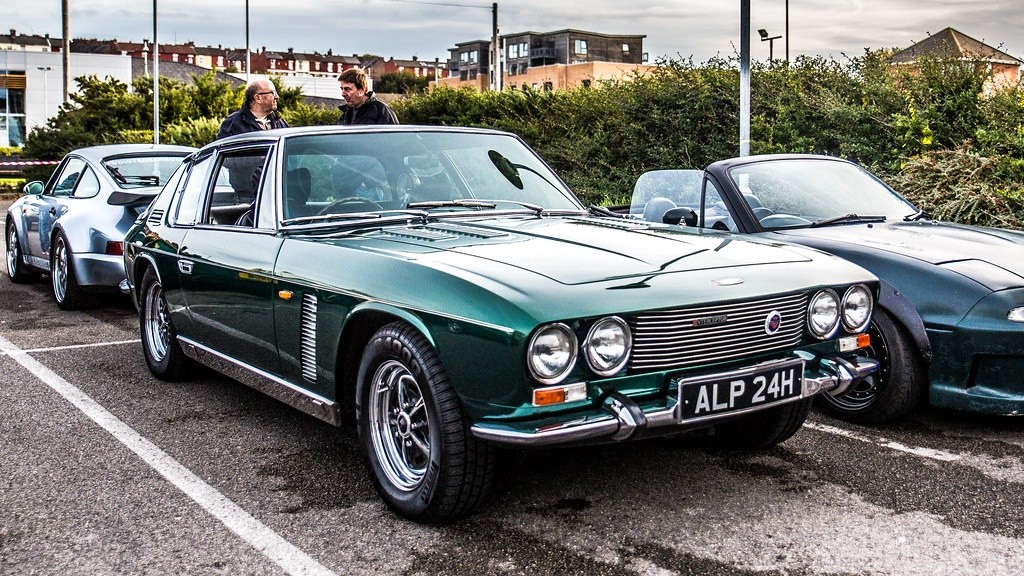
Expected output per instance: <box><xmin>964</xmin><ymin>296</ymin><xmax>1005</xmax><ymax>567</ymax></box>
<box><xmin>208</xmin><ymin>199</ymin><xmax>391</xmax><ymax>228</ymax></box>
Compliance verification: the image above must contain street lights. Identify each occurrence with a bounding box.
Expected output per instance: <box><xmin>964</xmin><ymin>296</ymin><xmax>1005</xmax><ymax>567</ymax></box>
<box><xmin>757</xmin><ymin>28</ymin><xmax>782</xmax><ymax>65</ymax></box>
<box><xmin>37</xmin><ymin>66</ymin><xmax>52</xmax><ymax>132</ymax></box>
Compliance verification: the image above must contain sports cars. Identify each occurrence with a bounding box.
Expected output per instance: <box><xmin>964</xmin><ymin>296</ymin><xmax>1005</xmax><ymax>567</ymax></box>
<box><xmin>603</xmin><ymin>150</ymin><xmax>1024</xmax><ymax>427</ymax></box>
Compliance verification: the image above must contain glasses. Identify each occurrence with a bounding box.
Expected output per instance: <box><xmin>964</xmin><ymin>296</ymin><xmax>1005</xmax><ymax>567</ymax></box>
<box><xmin>253</xmin><ymin>90</ymin><xmax>276</xmax><ymax>97</ymax></box>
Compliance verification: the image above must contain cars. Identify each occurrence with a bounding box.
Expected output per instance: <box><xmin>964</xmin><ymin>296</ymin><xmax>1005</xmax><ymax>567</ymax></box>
<box><xmin>118</xmin><ymin>120</ymin><xmax>884</xmax><ymax>528</ymax></box>
<box><xmin>3</xmin><ymin>141</ymin><xmax>236</xmax><ymax>311</ymax></box>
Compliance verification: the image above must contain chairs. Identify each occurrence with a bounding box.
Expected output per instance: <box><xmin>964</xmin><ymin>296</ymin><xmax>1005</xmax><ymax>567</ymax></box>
<box><xmin>727</xmin><ymin>194</ymin><xmax>769</xmax><ymax>235</ymax></box>
<box><xmin>643</xmin><ymin>197</ymin><xmax>683</xmax><ymax>227</ymax></box>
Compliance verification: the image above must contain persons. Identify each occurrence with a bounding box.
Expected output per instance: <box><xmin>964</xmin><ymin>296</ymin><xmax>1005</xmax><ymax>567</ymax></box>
<box><xmin>329</xmin><ymin>68</ymin><xmax>404</xmax><ymax>202</ymax></box>
<box><xmin>217</xmin><ymin>77</ymin><xmax>303</xmax><ymax>206</ymax></box>
